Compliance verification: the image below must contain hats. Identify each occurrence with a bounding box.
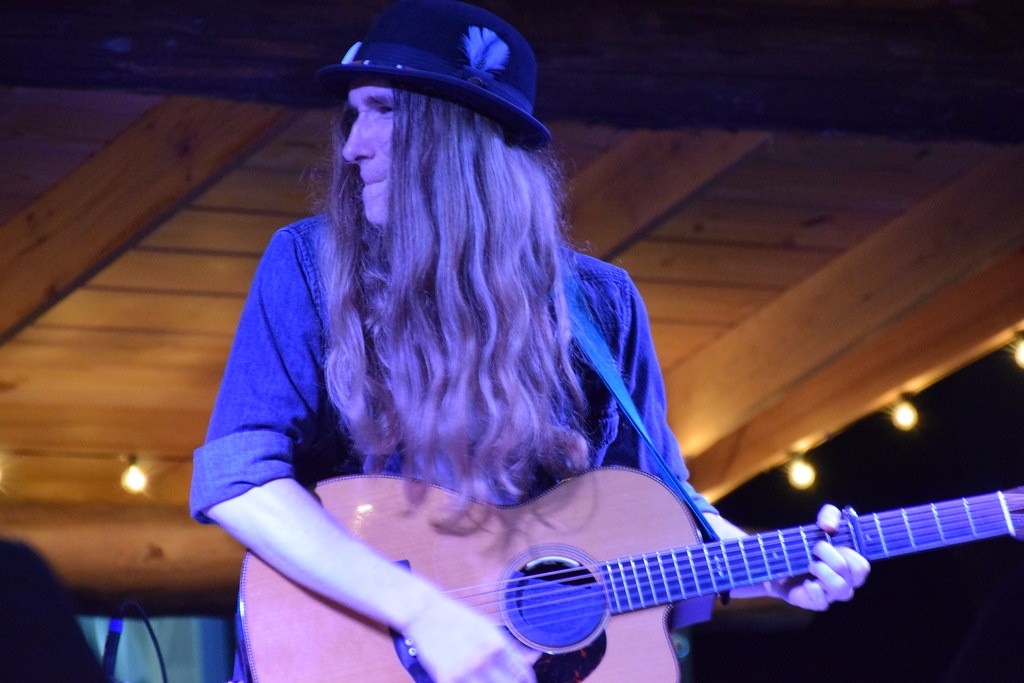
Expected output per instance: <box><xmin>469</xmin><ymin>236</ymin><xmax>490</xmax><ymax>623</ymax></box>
<box><xmin>313</xmin><ymin>0</ymin><xmax>551</xmax><ymax>154</ymax></box>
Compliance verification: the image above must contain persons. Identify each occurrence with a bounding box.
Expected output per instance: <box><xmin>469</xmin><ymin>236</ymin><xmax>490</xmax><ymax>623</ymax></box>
<box><xmin>191</xmin><ymin>1</ymin><xmax>871</xmax><ymax>682</ymax></box>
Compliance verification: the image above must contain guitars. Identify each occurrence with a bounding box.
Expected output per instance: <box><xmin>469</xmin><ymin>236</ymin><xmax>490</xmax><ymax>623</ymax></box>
<box><xmin>232</xmin><ymin>463</ymin><xmax>1023</xmax><ymax>682</ymax></box>
